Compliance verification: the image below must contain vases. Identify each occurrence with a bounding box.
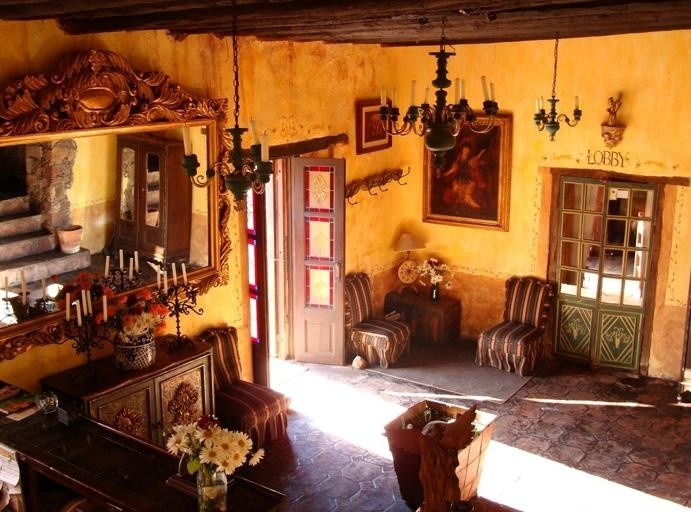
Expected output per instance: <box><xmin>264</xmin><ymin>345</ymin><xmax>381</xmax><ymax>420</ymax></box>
<box><xmin>113</xmin><ymin>336</ymin><xmax>158</xmax><ymax>371</ymax></box>
<box><xmin>197</xmin><ymin>467</ymin><xmax>228</xmax><ymax>512</ymax></box>
<box><xmin>432</xmin><ymin>285</ymin><xmax>440</xmax><ymax>300</ymax></box>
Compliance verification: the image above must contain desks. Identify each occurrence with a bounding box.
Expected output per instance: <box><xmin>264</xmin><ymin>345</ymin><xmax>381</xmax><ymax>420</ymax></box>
<box><xmin>385</xmin><ymin>291</ymin><xmax>460</xmax><ymax>347</ymax></box>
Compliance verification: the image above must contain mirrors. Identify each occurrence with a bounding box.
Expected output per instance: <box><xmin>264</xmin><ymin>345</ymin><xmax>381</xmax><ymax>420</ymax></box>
<box><xmin>1</xmin><ymin>50</ymin><xmax>234</xmax><ymax>362</ymax></box>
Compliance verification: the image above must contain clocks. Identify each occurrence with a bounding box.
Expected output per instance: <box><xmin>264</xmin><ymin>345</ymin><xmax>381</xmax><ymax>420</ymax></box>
<box><xmin>397</xmin><ymin>260</ymin><xmax>418</xmax><ymax>285</ymax></box>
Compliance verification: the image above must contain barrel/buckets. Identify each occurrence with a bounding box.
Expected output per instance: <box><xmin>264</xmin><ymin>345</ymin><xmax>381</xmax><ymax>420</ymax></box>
<box><xmin>56</xmin><ymin>224</ymin><xmax>83</xmax><ymax>254</ymax></box>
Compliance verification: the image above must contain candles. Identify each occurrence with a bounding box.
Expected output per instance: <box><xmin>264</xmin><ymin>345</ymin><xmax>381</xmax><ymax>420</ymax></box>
<box><xmin>65</xmin><ymin>289</ymin><xmax>110</xmax><ymax>327</ymax></box>
<box><xmin>102</xmin><ymin>250</ymin><xmax>143</xmax><ymax>288</ymax></box>
<box><xmin>5</xmin><ymin>270</ymin><xmax>48</xmax><ymax>308</ymax></box>
<box><xmin>156</xmin><ymin>262</ymin><xmax>190</xmax><ymax>296</ymax></box>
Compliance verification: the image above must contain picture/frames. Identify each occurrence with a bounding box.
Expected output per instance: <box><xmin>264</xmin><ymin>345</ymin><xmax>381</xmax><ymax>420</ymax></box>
<box><xmin>354</xmin><ymin>99</ymin><xmax>393</xmax><ymax>157</ymax></box>
<box><xmin>421</xmin><ymin>110</ymin><xmax>513</xmax><ymax>234</ymax></box>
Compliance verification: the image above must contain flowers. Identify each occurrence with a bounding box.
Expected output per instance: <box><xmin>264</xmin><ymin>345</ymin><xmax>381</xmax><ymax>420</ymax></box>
<box><xmin>417</xmin><ymin>258</ymin><xmax>455</xmax><ymax>290</ymax></box>
<box><xmin>168</xmin><ymin>421</ymin><xmax>267</xmax><ymax>476</ymax></box>
<box><xmin>96</xmin><ymin>286</ymin><xmax>168</xmax><ymax>339</ymax></box>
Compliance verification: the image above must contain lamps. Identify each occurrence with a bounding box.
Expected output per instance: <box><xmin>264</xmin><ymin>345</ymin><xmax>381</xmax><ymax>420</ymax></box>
<box><xmin>376</xmin><ymin>14</ymin><xmax>498</xmax><ymax>175</ymax></box>
<box><xmin>535</xmin><ymin>31</ymin><xmax>582</xmax><ymax>143</ymax></box>
<box><xmin>178</xmin><ymin>4</ymin><xmax>276</xmax><ymax>213</ymax></box>
<box><xmin>394</xmin><ymin>232</ymin><xmax>419</xmax><ymax>297</ymax></box>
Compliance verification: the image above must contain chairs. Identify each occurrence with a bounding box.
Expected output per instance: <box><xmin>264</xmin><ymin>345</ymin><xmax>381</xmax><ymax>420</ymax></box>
<box><xmin>344</xmin><ymin>273</ymin><xmax>411</xmax><ymax>369</ymax></box>
<box><xmin>476</xmin><ymin>275</ymin><xmax>557</xmax><ymax>377</ymax></box>
<box><xmin>203</xmin><ymin>326</ymin><xmax>288</xmax><ymax>449</ymax></box>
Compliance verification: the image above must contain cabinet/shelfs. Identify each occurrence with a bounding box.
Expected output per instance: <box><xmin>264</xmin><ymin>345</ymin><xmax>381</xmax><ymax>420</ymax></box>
<box><xmin>112</xmin><ymin>136</ymin><xmax>192</xmax><ymax>273</ymax></box>
<box><xmin>0</xmin><ymin>381</ymin><xmax>289</xmax><ymax>512</ymax></box>
<box><xmin>40</xmin><ymin>332</ymin><xmax>216</xmax><ymax>448</ymax></box>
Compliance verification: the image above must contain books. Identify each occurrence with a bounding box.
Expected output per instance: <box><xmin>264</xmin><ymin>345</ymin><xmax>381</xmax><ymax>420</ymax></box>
<box><xmin>0</xmin><ymin>394</ymin><xmax>34</xmax><ymax>415</ymax></box>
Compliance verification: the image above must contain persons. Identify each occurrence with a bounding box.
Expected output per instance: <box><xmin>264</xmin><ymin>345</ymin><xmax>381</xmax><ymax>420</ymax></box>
<box><xmin>441</xmin><ymin>140</ymin><xmax>486</xmax><ymax>208</ymax></box>
